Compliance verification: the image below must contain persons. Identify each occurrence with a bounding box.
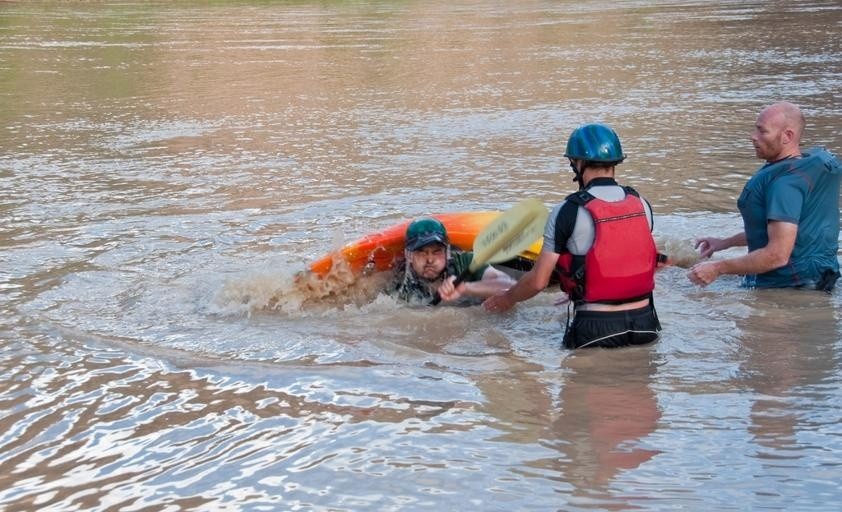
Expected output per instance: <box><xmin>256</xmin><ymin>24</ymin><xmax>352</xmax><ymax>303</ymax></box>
<box><xmin>686</xmin><ymin>101</ymin><xmax>842</xmax><ymax>292</ymax></box>
<box><xmin>481</xmin><ymin>124</ymin><xmax>662</xmax><ymax>350</ymax></box>
<box><xmin>378</xmin><ymin>218</ymin><xmax>518</xmax><ymax>306</ymax></box>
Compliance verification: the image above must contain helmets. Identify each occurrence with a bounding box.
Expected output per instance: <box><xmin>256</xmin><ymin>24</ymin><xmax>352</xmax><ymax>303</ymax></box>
<box><xmin>564</xmin><ymin>123</ymin><xmax>623</xmax><ymax>161</ymax></box>
<box><xmin>405</xmin><ymin>217</ymin><xmax>448</xmax><ymax>251</ymax></box>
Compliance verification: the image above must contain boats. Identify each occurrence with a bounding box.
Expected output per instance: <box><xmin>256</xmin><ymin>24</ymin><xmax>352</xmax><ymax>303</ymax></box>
<box><xmin>292</xmin><ymin>209</ymin><xmax>558</xmax><ymax>292</ymax></box>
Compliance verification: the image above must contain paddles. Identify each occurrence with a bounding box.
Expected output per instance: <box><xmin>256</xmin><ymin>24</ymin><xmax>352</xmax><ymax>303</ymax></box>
<box><xmin>429</xmin><ymin>197</ymin><xmax>553</xmax><ymax>305</ymax></box>
